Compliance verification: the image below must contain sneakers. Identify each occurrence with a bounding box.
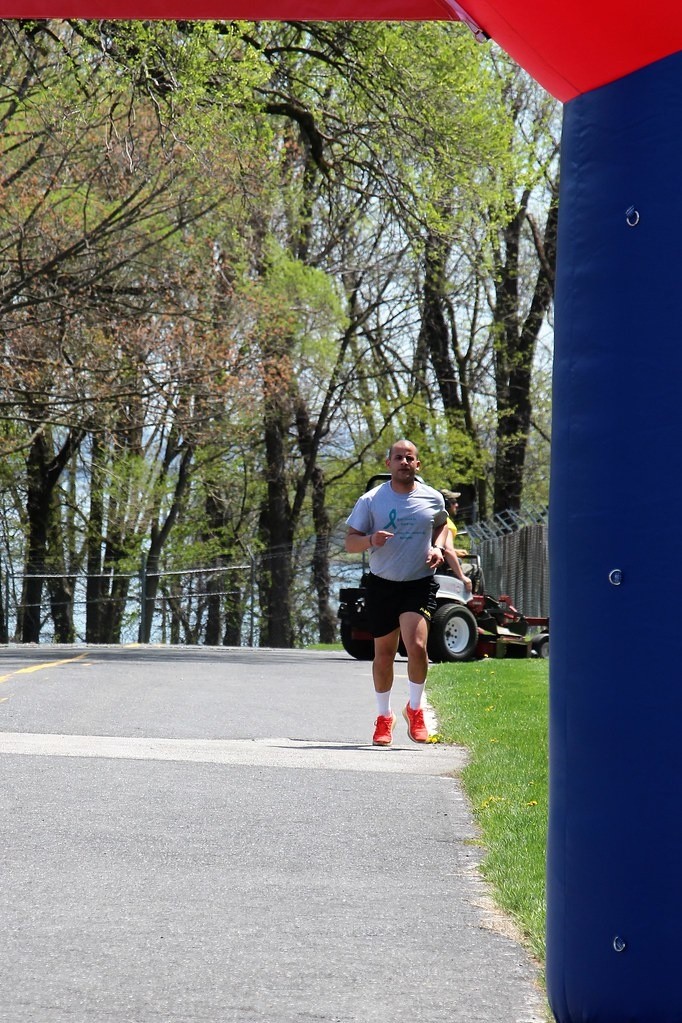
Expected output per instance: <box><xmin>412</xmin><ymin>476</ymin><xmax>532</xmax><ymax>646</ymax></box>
<box><xmin>401</xmin><ymin>698</ymin><xmax>429</xmax><ymax>743</ymax></box>
<box><xmin>372</xmin><ymin>708</ymin><xmax>397</xmax><ymax>746</ymax></box>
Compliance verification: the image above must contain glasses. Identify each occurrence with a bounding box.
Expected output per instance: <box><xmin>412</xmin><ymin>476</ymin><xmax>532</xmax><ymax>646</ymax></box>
<box><xmin>448</xmin><ymin>498</ymin><xmax>457</xmax><ymax>503</ymax></box>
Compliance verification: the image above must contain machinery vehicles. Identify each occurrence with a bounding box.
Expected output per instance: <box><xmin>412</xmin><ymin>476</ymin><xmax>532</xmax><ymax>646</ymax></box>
<box><xmin>337</xmin><ymin>472</ymin><xmax>550</xmax><ymax>663</ymax></box>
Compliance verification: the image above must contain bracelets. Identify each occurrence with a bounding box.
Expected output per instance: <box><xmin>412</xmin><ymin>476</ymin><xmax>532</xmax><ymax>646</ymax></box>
<box><xmin>434</xmin><ymin>545</ymin><xmax>445</xmax><ymax>556</ymax></box>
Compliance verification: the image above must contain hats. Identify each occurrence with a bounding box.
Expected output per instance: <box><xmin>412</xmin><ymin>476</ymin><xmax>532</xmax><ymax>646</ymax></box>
<box><xmin>440</xmin><ymin>488</ymin><xmax>461</xmax><ymax>498</ymax></box>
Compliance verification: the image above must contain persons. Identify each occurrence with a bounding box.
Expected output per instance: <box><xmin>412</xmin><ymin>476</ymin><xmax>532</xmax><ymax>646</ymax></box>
<box><xmin>435</xmin><ymin>488</ymin><xmax>485</xmax><ymax>595</ymax></box>
<box><xmin>344</xmin><ymin>439</ymin><xmax>450</xmax><ymax>747</ymax></box>
